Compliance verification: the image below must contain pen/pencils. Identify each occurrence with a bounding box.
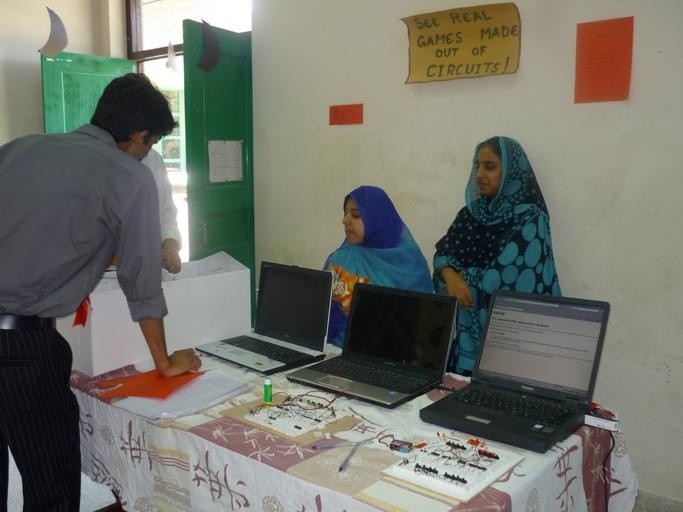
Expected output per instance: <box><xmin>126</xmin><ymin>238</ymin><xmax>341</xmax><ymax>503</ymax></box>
<box><xmin>339</xmin><ymin>445</ymin><xmax>359</xmax><ymax>472</ymax></box>
<box><xmin>312</xmin><ymin>441</ymin><xmax>374</xmax><ymax>449</ymax></box>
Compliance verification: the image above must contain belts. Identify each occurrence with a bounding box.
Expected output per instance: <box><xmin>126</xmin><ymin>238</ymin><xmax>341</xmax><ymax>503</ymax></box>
<box><xmin>0</xmin><ymin>314</ymin><xmax>53</xmax><ymax>331</ymax></box>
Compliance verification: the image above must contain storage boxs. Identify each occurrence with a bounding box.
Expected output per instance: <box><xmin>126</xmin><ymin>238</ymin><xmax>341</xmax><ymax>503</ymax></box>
<box><xmin>55</xmin><ymin>249</ymin><xmax>251</xmax><ymax>377</ymax></box>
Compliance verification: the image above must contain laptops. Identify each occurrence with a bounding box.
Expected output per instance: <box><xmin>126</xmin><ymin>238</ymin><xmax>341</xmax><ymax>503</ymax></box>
<box><xmin>195</xmin><ymin>261</ymin><xmax>334</xmax><ymax>375</ymax></box>
<box><xmin>286</xmin><ymin>282</ymin><xmax>457</xmax><ymax>409</ymax></box>
<box><xmin>420</xmin><ymin>290</ymin><xmax>610</xmax><ymax>454</ymax></box>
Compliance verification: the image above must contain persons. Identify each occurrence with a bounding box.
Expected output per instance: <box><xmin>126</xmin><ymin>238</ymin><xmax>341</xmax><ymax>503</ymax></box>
<box><xmin>322</xmin><ymin>185</ymin><xmax>434</xmax><ymax>349</ymax></box>
<box><xmin>0</xmin><ymin>72</ymin><xmax>201</xmax><ymax>512</ymax></box>
<box><xmin>109</xmin><ymin>147</ymin><xmax>183</xmax><ymax>275</ymax></box>
<box><xmin>434</xmin><ymin>137</ymin><xmax>563</xmax><ymax>376</ymax></box>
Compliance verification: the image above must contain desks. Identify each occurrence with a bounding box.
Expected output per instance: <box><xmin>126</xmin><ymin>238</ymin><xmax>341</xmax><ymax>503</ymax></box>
<box><xmin>69</xmin><ymin>343</ymin><xmax>641</xmax><ymax>512</ymax></box>
